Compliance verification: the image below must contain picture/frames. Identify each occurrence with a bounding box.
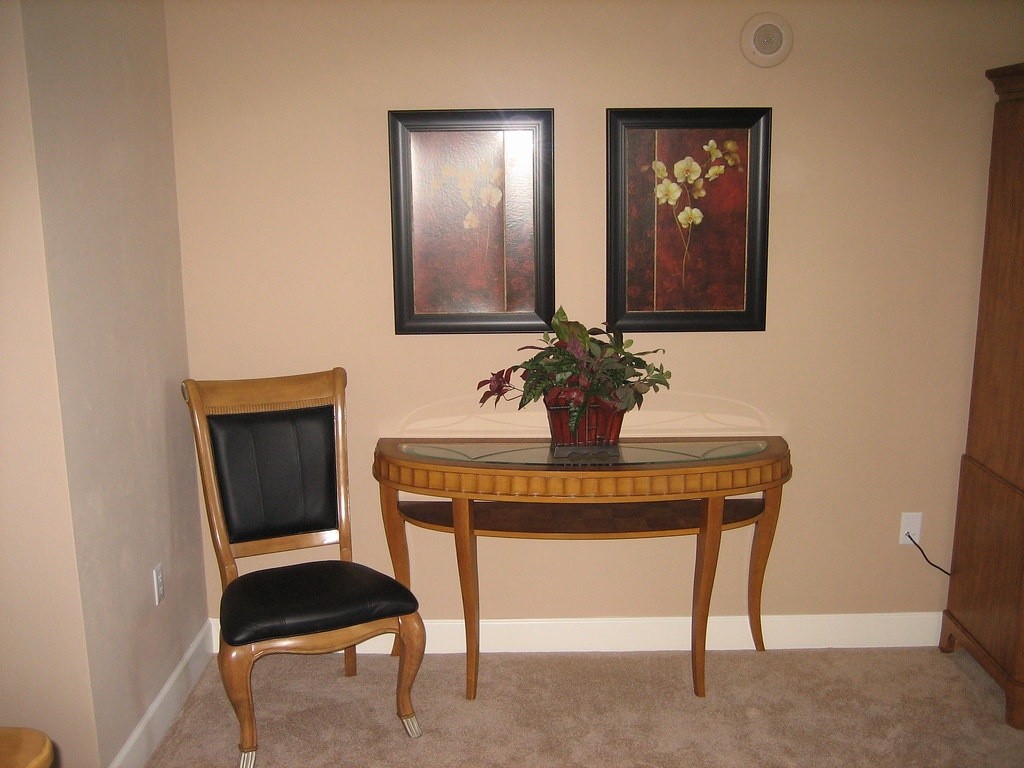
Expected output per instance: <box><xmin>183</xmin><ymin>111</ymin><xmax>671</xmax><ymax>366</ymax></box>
<box><xmin>607</xmin><ymin>107</ymin><xmax>774</xmax><ymax>335</ymax></box>
<box><xmin>386</xmin><ymin>109</ymin><xmax>558</xmax><ymax>337</ymax></box>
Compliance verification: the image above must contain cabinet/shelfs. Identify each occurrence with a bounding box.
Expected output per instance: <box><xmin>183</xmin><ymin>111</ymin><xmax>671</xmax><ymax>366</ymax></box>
<box><xmin>939</xmin><ymin>63</ymin><xmax>1024</xmax><ymax>732</ymax></box>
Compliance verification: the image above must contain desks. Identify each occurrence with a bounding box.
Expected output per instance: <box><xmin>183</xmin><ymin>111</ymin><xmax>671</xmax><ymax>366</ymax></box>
<box><xmin>372</xmin><ymin>434</ymin><xmax>795</xmax><ymax>699</ymax></box>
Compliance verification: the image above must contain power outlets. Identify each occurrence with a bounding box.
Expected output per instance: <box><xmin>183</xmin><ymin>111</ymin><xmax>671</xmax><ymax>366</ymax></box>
<box><xmin>899</xmin><ymin>512</ymin><xmax>923</xmax><ymax>545</ymax></box>
<box><xmin>152</xmin><ymin>562</ymin><xmax>165</xmax><ymax>606</ymax></box>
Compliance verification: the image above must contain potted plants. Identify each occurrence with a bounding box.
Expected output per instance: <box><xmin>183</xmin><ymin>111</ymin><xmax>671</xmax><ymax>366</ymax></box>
<box><xmin>473</xmin><ymin>306</ymin><xmax>674</xmax><ymax>451</ymax></box>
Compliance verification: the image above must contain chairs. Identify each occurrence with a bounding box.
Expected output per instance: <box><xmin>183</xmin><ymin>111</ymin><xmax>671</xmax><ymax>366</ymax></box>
<box><xmin>180</xmin><ymin>368</ymin><xmax>429</xmax><ymax>768</ymax></box>
